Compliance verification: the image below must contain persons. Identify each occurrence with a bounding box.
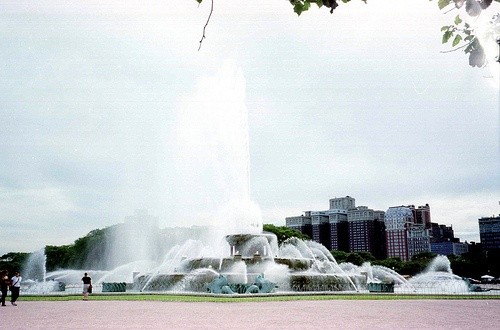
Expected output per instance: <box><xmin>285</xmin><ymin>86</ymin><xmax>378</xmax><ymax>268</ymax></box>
<box><xmin>81</xmin><ymin>273</ymin><xmax>92</xmax><ymax>300</ymax></box>
<box><xmin>0</xmin><ymin>270</ymin><xmax>10</xmax><ymax>307</ymax></box>
<box><xmin>8</xmin><ymin>271</ymin><xmax>22</xmax><ymax>306</ymax></box>
